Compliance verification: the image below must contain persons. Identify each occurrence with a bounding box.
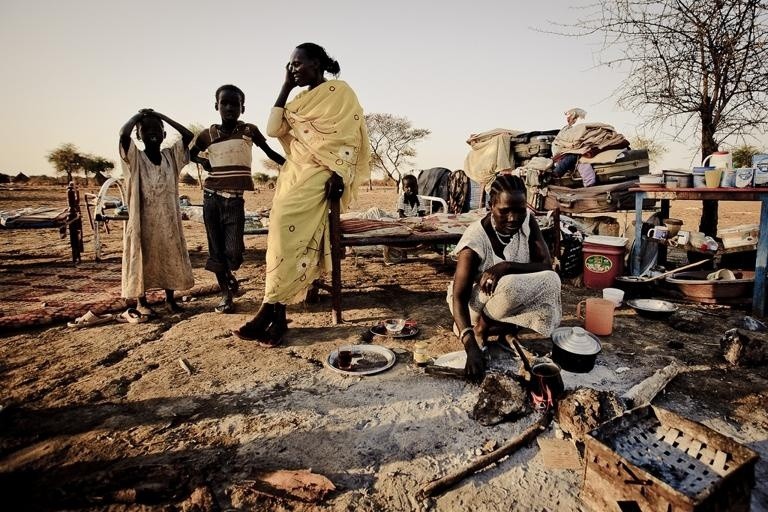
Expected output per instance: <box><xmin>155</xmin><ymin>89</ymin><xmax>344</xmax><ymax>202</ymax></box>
<box><xmin>232</xmin><ymin>42</ymin><xmax>371</xmax><ymax>343</ymax></box>
<box><xmin>397</xmin><ymin>175</ymin><xmax>431</xmax><ymax>259</ymax></box>
<box><xmin>446</xmin><ymin>174</ymin><xmax>562</xmax><ymax>386</ymax></box>
<box><xmin>118</xmin><ymin>109</ymin><xmax>195</xmax><ymax>317</ymax></box>
<box><xmin>190</xmin><ymin>85</ymin><xmax>286</xmax><ymax>312</ymax></box>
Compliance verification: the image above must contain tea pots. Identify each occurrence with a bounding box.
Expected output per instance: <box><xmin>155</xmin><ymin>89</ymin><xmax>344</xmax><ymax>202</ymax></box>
<box><xmin>576</xmin><ymin>298</ymin><xmax>616</xmax><ymax>336</ymax></box>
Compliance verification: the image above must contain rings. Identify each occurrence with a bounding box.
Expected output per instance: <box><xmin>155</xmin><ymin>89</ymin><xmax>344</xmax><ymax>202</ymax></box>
<box><xmin>487</xmin><ymin>279</ymin><xmax>492</xmax><ymax>284</ymax></box>
<box><xmin>339</xmin><ymin>189</ymin><xmax>342</xmax><ymax>191</ymax></box>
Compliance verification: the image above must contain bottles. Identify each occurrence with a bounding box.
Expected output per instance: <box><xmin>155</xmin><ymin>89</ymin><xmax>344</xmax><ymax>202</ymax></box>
<box><xmin>529</xmin><ymin>363</ymin><xmax>564</xmax><ymax>408</ymax></box>
<box><xmin>412</xmin><ymin>343</ymin><xmax>428</xmax><ymax>367</ymax></box>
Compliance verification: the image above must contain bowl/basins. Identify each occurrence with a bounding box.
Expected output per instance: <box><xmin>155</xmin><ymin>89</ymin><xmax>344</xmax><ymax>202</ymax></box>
<box><xmin>665</xmin><ymin>270</ymin><xmax>756</xmax><ymax>300</ymax></box>
<box><xmin>383</xmin><ymin>319</ymin><xmax>407</xmax><ymax>332</ymax></box>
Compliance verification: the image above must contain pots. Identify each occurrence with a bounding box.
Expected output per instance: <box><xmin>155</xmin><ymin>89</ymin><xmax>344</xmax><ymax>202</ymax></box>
<box><xmin>550</xmin><ymin>327</ymin><xmax>603</xmax><ymax>374</ymax></box>
<box><xmin>626</xmin><ymin>298</ymin><xmax>679</xmax><ymax>320</ymax></box>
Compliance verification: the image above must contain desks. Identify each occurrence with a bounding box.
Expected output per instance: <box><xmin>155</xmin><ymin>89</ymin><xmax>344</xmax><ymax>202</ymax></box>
<box><xmin>628</xmin><ymin>187</ymin><xmax>768</xmax><ymax>322</ymax></box>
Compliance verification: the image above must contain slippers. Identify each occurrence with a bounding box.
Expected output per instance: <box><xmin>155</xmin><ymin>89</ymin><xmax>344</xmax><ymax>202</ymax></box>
<box><xmin>67</xmin><ymin>310</ymin><xmax>113</xmax><ymax>327</ymax></box>
<box><xmin>233</xmin><ymin>318</ymin><xmax>270</xmax><ymax>341</ymax></box>
<box><xmin>115</xmin><ymin>308</ymin><xmax>148</xmax><ymax>324</ymax></box>
<box><xmin>496</xmin><ymin>339</ymin><xmax>539</xmax><ymax>358</ymax></box>
<box><xmin>259</xmin><ymin>324</ymin><xmax>285</xmax><ymax>347</ymax></box>
<box><xmin>227</xmin><ymin>275</ymin><xmax>239</xmax><ymax>291</ymax></box>
<box><xmin>214</xmin><ymin>295</ymin><xmax>234</xmax><ymax>314</ymax></box>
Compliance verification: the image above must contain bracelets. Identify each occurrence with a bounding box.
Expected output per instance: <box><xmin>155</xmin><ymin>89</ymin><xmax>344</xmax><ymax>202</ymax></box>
<box><xmin>460</xmin><ymin>327</ymin><xmax>475</xmax><ymax>344</ymax></box>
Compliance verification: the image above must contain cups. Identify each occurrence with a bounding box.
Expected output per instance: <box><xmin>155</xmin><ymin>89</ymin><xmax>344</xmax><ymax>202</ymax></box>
<box><xmin>648</xmin><ymin>224</ymin><xmax>668</xmax><ymax>239</ymax></box>
<box><xmin>336</xmin><ymin>342</ymin><xmax>353</xmax><ymax>370</ymax></box>
<box><xmin>663</xmin><ymin>219</ymin><xmax>683</xmax><ymax>235</ymax></box>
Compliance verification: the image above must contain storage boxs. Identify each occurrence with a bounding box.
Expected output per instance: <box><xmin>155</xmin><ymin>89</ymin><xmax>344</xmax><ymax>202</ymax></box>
<box><xmin>694</xmin><ymin>172</ymin><xmax>711</xmax><ymax>188</ymax></box>
<box><xmin>735</xmin><ymin>166</ymin><xmax>755</xmax><ymax>188</ymax></box>
<box><xmin>752</xmin><ymin>154</ymin><xmax>768</xmax><ymax>188</ymax></box>
<box><xmin>663</xmin><ymin>168</ymin><xmax>692</xmax><ymax>188</ymax></box>
<box><xmin>581</xmin><ymin>234</ymin><xmax>629</xmax><ymax>288</ymax></box>
<box><xmin>716</xmin><ymin>222</ymin><xmax>761</xmax><ymax>249</ymax></box>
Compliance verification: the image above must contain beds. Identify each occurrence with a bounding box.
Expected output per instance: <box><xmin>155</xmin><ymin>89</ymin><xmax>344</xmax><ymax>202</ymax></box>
<box><xmin>1</xmin><ymin>180</ymin><xmax>84</xmax><ymax>261</ymax></box>
<box><xmin>308</xmin><ymin>171</ymin><xmax>561</xmax><ymax>330</ymax></box>
<box><xmin>95</xmin><ymin>178</ymin><xmax>271</xmax><ymax>261</ymax></box>
<box><xmin>337</xmin><ymin>194</ymin><xmax>450</xmax><ymax>263</ymax></box>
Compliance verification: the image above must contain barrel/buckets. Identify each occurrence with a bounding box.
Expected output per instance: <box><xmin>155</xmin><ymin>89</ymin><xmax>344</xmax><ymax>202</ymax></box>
<box><xmin>691</xmin><ymin>165</ymin><xmax>717</xmax><ymax>188</ymax></box>
<box><xmin>706</xmin><ymin>269</ymin><xmax>736</xmax><ymax>281</ymax></box>
<box><xmin>708</xmin><ymin>151</ymin><xmax>732</xmax><ymax>168</ymax></box>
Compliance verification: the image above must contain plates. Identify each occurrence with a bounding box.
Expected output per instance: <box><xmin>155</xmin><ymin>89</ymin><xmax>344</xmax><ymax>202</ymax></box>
<box><xmin>326</xmin><ymin>344</ymin><xmax>397</xmax><ymax>378</ymax></box>
<box><xmin>433</xmin><ymin>350</ymin><xmax>468</xmax><ymax>370</ymax></box>
<box><xmin>369</xmin><ymin>324</ymin><xmax>418</xmax><ymax>338</ymax></box>
